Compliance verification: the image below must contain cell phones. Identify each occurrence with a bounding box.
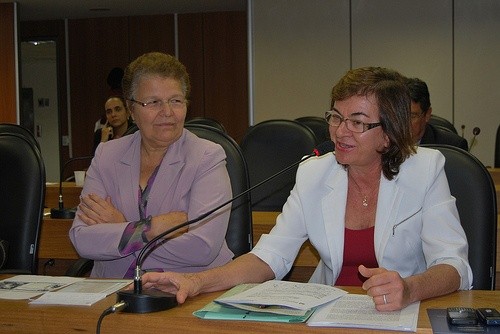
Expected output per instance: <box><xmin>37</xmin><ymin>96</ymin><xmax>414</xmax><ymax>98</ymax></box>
<box><xmin>445</xmin><ymin>306</ymin><xmax>478</xmax><ymax>326</ymax></box>
<box><xmin>477</xmin><ymin>307</ymin><xmax>500</xmax><ymax>325</ymax></box>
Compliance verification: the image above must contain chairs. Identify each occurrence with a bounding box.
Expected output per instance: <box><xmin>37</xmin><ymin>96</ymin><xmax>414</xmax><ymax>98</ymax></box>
<box><xmin>124</xmin><ymin>114</ymin><xmax>497</xmax><ymax>290</ymax></box>
<box><xmin>0</xmin><ymin>123</ymin><xmax>46</xmax><ymax>276</ymax></box>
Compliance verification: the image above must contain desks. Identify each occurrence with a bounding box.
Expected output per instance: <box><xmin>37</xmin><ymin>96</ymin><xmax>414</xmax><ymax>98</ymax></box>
<box><xmin>37</xmin><ymin>208</ymin><xmax>321</xmax><ymax>276</ymax></box>
<box><xmin>0</xmin><ymin>273</ymin><xmax>500</xmax><ymax>334</ymax></box>
<box><xmin>44</xmin><ymin>182</ymin><xmax>84</xmax><ymax>210</ymax></box>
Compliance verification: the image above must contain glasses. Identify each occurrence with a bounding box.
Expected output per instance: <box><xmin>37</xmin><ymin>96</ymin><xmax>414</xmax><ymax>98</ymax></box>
<box><xmin>324</xmin><ymin>111</ymin><xmax>380</xmax><ymax>134</ymax></box>
<box><xmin>128</xmin><ymin>98</ymin><xmax>188</xmax><ymax>109</ymax></box>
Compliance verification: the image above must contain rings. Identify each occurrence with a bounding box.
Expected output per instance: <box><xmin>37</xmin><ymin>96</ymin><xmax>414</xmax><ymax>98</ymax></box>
<box><xmin>384</xmin><ymin>296</ymin><xmax>387</xmax><ymax>304</ymax></box>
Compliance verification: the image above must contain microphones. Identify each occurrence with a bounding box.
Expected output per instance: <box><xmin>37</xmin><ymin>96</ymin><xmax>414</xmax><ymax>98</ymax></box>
<box><xmin>116</xmin><ymin>141</ymin><xmax>335</xmax><ymax>313</ymax></box>
<box><xmin>461</xmin><ymin>125</ymin><xmax>465</xmax><ymax>138</ymax></box>
<box><xmin>468</xmin><ymin>127</ymin><xmax>481</xmax><ymax>152</ymax></box>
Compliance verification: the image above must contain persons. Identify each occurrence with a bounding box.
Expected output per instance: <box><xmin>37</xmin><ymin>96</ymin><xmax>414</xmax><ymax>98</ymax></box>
<box><xmin>69</xmin><ymin>52</ymin><xmax>234</xmax><ymax>278</ymax></box>
<box><xmin>405</xmin><ymin>77</ymin><xmax>450</xmax><ymax>146</ymax></box>
<box><xmin>127</xmin><ymin>66</ymin><xmax>472</xmax><ymax>311</ymax></box>
<box><xmin>91</xmin><ymin>95</ymin><xmax>139</xmax><ymax>160</ymax></box>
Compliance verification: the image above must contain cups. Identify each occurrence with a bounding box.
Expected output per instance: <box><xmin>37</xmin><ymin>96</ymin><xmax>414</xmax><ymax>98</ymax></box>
<box><xmin>74</xmin><ymin>171</ymin><xmax>86</xmax><ymax>186</ymax></box>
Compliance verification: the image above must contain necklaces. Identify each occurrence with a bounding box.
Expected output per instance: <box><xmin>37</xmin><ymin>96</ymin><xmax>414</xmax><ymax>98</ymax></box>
<box><xmin>348</xmin><ymin>170</ymin><xmax>381</xmax><ymax>206</ymax></box>
